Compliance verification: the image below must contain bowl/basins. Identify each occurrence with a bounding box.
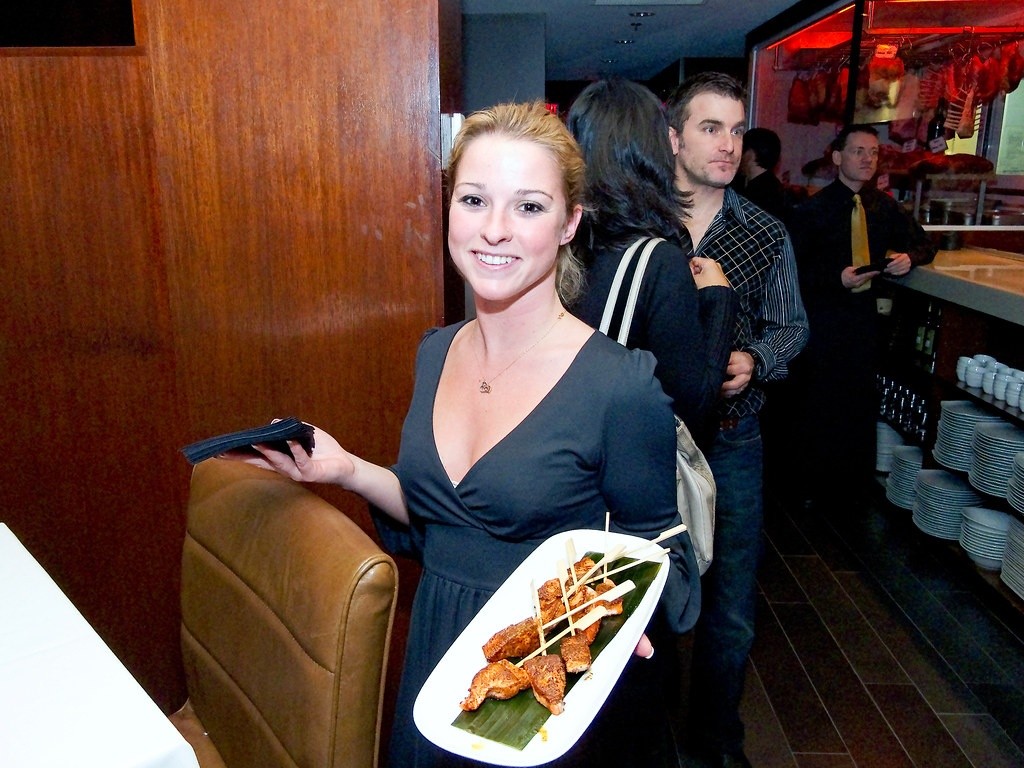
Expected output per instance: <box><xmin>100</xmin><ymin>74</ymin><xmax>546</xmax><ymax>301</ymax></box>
<box><xmin>956</xmin><ymin>354</ymin><xmax>1024</xmax><ymax>413</ymax></box>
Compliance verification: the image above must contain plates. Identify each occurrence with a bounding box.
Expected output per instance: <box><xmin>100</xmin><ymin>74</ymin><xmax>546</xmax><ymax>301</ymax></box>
<box><xmin>412</xmin><ymin>529</ymin><xmax>670</xmax><ymax>767</ymax></box>
<box><xmin>875</xmin><ymin>399</ymin><xmax>1024</xmax><ymax>602</ymax></box>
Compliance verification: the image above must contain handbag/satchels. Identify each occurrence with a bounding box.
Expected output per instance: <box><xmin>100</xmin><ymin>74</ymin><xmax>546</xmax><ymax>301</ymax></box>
<box><xmin>597</xmin><ymin>234</ymin><xmax>719</xmax><ymax>578</ymax></box>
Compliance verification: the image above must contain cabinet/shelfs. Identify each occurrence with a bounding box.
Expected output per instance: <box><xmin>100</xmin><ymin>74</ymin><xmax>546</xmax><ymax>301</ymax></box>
<box><xmin>875</xmin><ymin>267</ymin><xmax>1024</xmax><ymax>617</ymax></box>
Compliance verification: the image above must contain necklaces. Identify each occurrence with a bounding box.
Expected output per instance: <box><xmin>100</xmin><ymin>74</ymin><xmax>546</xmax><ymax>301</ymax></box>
<box><xmin>469</xmin><ymin>308</ymin><xmax>567</xmax><ymax>393</ymax></box>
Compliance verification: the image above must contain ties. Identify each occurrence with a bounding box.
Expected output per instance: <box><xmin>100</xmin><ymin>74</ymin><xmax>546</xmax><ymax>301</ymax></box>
<box><xmin>851</xmin><ymin>192</ymin><xmax>871</xmax><ymax>293</ymax></box>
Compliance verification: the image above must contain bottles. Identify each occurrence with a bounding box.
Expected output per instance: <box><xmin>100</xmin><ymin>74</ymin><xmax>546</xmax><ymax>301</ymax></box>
<box><xmin>915</xmin><ymin>301</ymin><xmax>944</xmax><ymax>356</ymax></box>
<box><xmin>925</xmin><ymin>96</ymin><xmax>946</xmax><ymax>151</ymax></box>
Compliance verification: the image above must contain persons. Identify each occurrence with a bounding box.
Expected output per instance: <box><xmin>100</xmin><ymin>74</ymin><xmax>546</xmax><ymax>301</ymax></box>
<box><xmin>564</xmin><ymin>72</ymin><xmax>935</xmax><ymax>688</ymax></box>
<box><xmin>231</xmin><ymin>104</ymin><xmax>703</xmax><ymax>768</ymax></box>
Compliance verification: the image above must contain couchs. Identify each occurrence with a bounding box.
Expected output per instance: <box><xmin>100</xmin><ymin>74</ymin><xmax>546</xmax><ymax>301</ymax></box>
<box><xmin>166</xmin><ymin>453</ymin><xmax>399</xmax><ymax>768</ymax></box>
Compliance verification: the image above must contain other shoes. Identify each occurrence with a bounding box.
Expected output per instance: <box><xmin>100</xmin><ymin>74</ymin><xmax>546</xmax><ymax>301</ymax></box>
<box><xmin>684</xmin><ymin>693</ymin><xmax>754</xmax><ymax>767</ymax></box>
